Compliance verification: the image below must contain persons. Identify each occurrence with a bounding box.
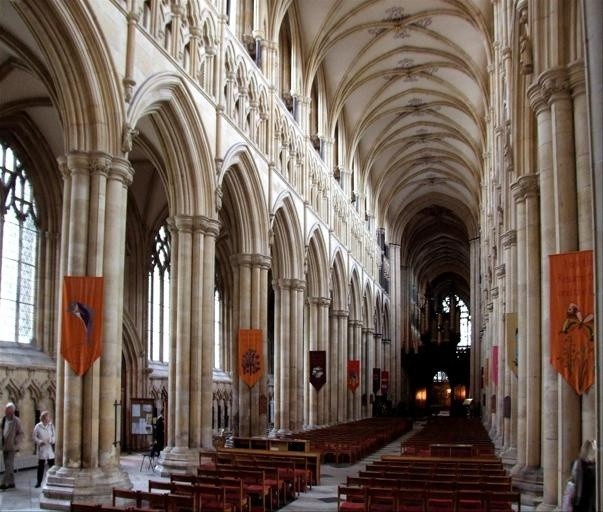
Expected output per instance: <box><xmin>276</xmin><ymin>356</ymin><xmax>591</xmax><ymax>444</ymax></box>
<box><xmin>32</xmin><ymin>411</ymin><xmax>55</xmax><ymax>487</ymax></box>
<box><xmin>568</xmin><ymin>439</ymin><xmax>597</xmax><ymax>511</ymax></box>
<box><xmin>151</xmin><ymin>409</ymin><xmax>163</xmax><ymax>455</ymax></box>
<box><xmin>0</xmin><ymin>402</ymin><xmax>23</xmax><ymax>490</ymax></box>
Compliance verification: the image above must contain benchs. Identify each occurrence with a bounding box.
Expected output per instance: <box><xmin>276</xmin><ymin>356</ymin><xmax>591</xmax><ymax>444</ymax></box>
<box><xmin>416</xmin><ymin>411</ymin><xmax>522</xmax><ymax>512</ymax></box>
